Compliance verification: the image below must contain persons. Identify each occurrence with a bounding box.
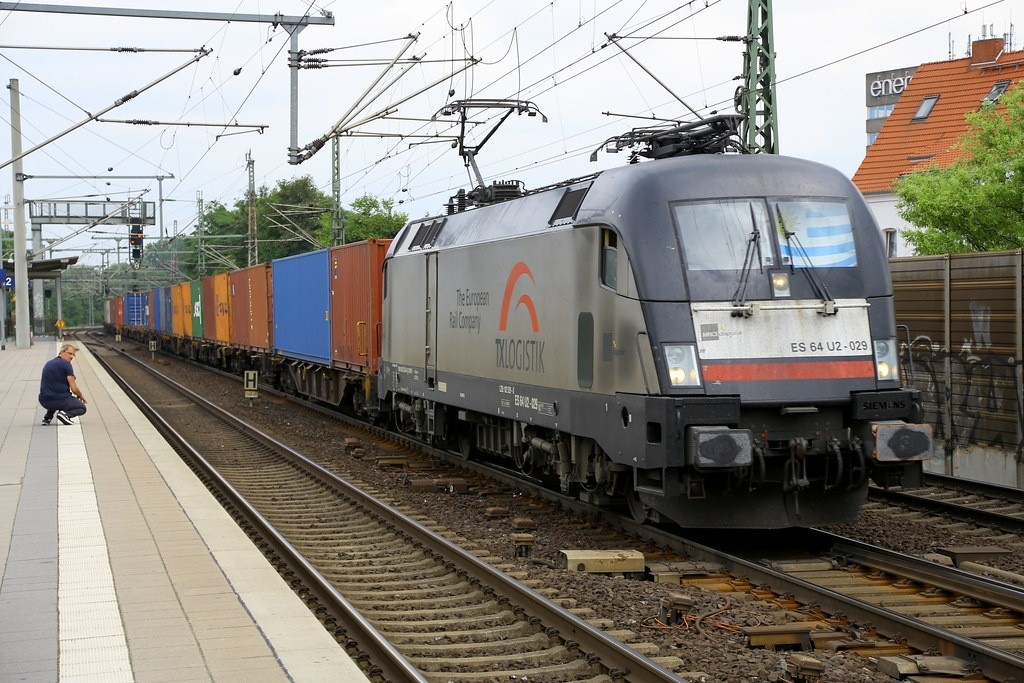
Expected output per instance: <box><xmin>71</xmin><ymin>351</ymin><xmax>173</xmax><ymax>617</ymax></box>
<box><xmin>38</xmin><ymin>344</ymin><xmax>87</xmax><ymax>426</ymax></box>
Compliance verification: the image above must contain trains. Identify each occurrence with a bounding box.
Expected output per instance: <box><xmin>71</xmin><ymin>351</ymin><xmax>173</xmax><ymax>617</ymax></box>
<box><xmin>99</xmin><ymin>97</ymin><xmax>937</xmax><ymax>531</ymax></box>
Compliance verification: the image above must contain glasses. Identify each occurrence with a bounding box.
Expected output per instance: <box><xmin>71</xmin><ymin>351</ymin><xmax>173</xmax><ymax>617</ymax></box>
<box><xmin>66</xmin><ymin>352</ymin><xmax>75</xmax><ymax>357</ymax></box>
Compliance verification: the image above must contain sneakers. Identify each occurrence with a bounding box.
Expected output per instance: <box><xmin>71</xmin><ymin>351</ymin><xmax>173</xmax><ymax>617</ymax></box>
<box><xmin>57</xmin><ymin>411</ymin><xmax>74</xmax><ymax>425</ymax></box>
<box><xmin>42</xmin><ymin>418</ymin><xmax>51</xmax><ymax>426</ymax></box>
